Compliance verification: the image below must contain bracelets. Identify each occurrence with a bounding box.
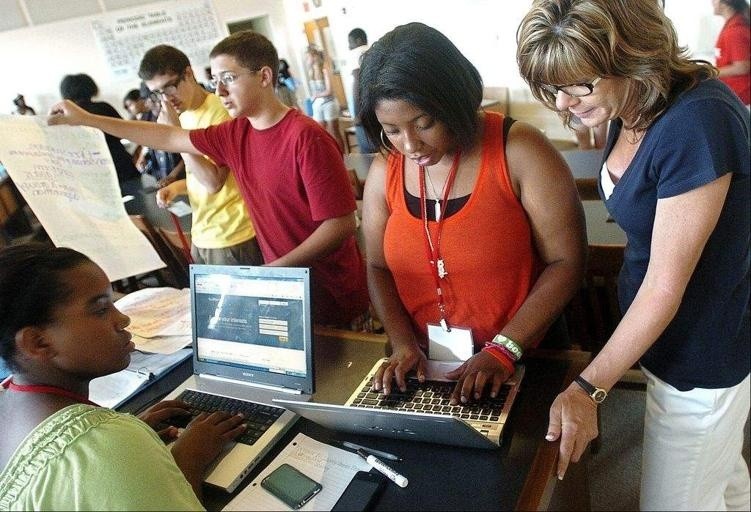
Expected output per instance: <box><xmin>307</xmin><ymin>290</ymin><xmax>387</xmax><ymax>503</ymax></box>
<box><xmin>478</xmin><ymin>330</ymin><xmax>523</xmax><ymax>374</ymax></box>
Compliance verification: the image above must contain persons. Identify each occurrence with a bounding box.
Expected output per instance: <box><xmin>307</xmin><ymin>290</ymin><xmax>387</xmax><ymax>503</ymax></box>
<box><xmin>0</xmin><ymin>241</ymin><xmax>247</xmax><ymax>512</ymax></box>
<box><xmin>49</xmin><ymin>34</ymin><xmax>374</xmax><ymax>331</ymax></box>
<box><xmin>516</xmin><ymin>1</ymin><xmax>751</xmax><ymax>512</ymax></box>
<box><xmin>355</xmin><ymin>23</ymin><xmax>592</xmax><ymax>405</ymax></box>
<box><xmin>710</xmin><ymin>2</ymin><xmax>751</xmax><ymax>112</ymax></box>
<box><xmin>12</xmin><ymin>93</ymin><xmax>37</xmax><ymax>114</ymax></box>
<box><xmin>348</xmin><ymin>28</ymin><xmax>378</xmax><ymax>153</ymax></box>
<box><xmin>305</xmin><ymin>50</ymin><xmax>345</xmax><ymax>154</ymax></box>
<box><xmin>139</xmin><ymin>44</ymin><xmax>265</xmax><ymax>266</ymax></box>
<box><xmin>276</xmin><ymin>60</ymin><xmax>300</xmax><ymax>110</ymax></box>
<box><xmin>60</xmin><ymin>73</ymin><xmax>187</xmax><ymax>214</ymax></box>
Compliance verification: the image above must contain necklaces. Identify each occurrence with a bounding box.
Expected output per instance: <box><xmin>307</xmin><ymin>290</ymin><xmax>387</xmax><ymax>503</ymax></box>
<box><xmin>425</xmin><ymin>162</ymin><xmax>452</xmax><ymax>229</ymax></box>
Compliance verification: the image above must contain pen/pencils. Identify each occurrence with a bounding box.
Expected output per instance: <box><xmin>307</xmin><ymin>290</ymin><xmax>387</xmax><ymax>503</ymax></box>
<box><xmin>336</xmin><ymin>440</ymin><xmax>405</xmax><ymax>461</ymax></box>
<box><xmin>357</xmin><ymin>448</ymin><xmax>409</xmax><ymax>488</ymax></box>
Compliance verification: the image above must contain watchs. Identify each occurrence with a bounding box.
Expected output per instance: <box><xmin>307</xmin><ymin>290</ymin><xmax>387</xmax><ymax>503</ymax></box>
<box><xmin>576</xmin><ymin>375</ymin><xmax>608</xmax><ymax>405</ymax></box>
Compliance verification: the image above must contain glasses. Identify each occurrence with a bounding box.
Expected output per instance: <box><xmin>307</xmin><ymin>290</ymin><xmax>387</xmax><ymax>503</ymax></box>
<box><xmin>147</xmin><ymin>74</ymin><xmax>180</xmax><ymax>100</ymax></box>
<box><xmin>533</xmin><ymin>77</ymin><xmax>601</xmax><ymax>96</ymax></box>
<box><xmin>209</xmin><ymin>71</ymin><xmax>254</xmax><ymax>88</ymax></box>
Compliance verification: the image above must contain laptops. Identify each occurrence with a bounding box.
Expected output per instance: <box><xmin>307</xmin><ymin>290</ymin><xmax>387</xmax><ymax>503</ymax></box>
<box><xmin>271</xmin><ymin>356</ymin><xmax>525</xmax><ymax>450</ymax></box>
<box><xmin>154</xmin><ymin>264</ymin><xmax>316</xmax><ymax>494</ymax></box>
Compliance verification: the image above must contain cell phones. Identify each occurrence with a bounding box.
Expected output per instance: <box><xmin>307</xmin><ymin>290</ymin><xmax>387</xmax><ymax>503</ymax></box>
<box><xmin>330</xmin><ymin>471</ymin><xmax>387</xmax><ymax>512</ymax></box>
<box><xmin>260</xmin><ymin>464</ymin><xmax>323</xmax><ymax>510</ymax></box>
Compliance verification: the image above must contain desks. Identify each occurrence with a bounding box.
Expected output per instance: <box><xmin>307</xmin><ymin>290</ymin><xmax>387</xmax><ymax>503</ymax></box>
<box><xmin>479</xmin><ymin>98</ymin><xmax>500</xmax><ymax>109</ymax></box>
<box><xmin>343</xmin><ymin>148</ymin><xmax>604</xmax><ymax>194</ymax></box>
<box><xmin>354</xmin><ymin>198</ymin><xmax>647</xmax><ymax>383</ymax></box>
<box><xmin>112</xmin><ymin>292</ymin><xmax>592</xmax><ymax>511</ymax></box>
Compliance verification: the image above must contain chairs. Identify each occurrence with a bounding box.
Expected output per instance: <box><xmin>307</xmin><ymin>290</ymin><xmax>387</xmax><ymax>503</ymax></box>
<box><xmin>122</xmin><ymin>213</ymin><xmax>191</xmax><ymax>293</ymax></box>
<box><xmin>344</xmin><ymin>125</ymin><xmax>357</xmax><ymax>153</ymax></box>
